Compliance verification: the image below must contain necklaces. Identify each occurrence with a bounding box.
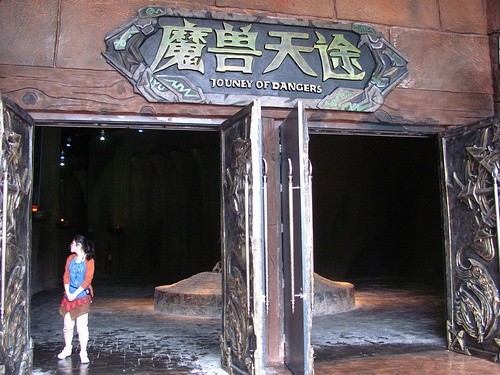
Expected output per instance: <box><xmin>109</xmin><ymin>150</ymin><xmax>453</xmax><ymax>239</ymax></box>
<box><xmin>73</xmin><ymin>253</ymin><xmax>86</xmax><ymax>287</ymax></box>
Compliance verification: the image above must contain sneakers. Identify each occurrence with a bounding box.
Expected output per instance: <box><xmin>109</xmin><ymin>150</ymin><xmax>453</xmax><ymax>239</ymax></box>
<box><xmin>79</xmin><ymin>352</ymin><xmax>89</xmax><ymax>363</ymax></box>
<box><xmin>57</xmin><ymin>348</ymin><xmax>72</xmax><ymax>359</ymax></box>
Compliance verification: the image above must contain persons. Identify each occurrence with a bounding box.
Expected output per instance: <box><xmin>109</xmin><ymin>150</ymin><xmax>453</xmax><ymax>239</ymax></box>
<box><xmin>103</xmin><ymin>244</ymin><xmax>113</xmax><ymax>274</ymax></box>
<box><xmin>57</xmin><ymin>234</ymin><xmax>96</xmax><ymax>364</ymax></box>
